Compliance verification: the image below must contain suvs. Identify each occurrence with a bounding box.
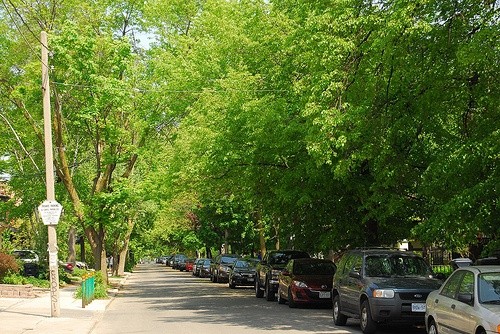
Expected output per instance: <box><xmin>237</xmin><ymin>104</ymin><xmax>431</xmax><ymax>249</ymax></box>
<box><xmin>12</xmin><ymin>249</ymin><xmax>41</xmax><ymax>276</ymax></box>
<box><xmin>331</xmin><ymin>247</ymin><xmax>450</xmax><ymax>334</ymax></box>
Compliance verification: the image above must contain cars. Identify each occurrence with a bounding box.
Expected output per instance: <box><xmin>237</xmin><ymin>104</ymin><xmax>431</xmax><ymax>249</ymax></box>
<box><xmin>228</xmin><ymin>258</ymin><xmax>261</xmax><ymax>289</ymax></box>
<box><xmin>158</xmin><ymin>253</ymin><xmax>239</xmax><ymax>283</ymax></box>
<box><xmin>278</xmin><ymin>257</ymin><xmax>338</xmax><ymax>309</ymax></box>
<box><xmin>43</xmin><ymin>258</ymin><xmax>88</xmax><ymax>273</ymax></box>
<box><xmin>254</xmin><ymin>249</ymin><xmax>312</xmax><ymax>302</ymax></box>
<box><xmin>424</xmin><ymin>265</ymin><xmax>500</xmax><ymax>334</ymax></box>
